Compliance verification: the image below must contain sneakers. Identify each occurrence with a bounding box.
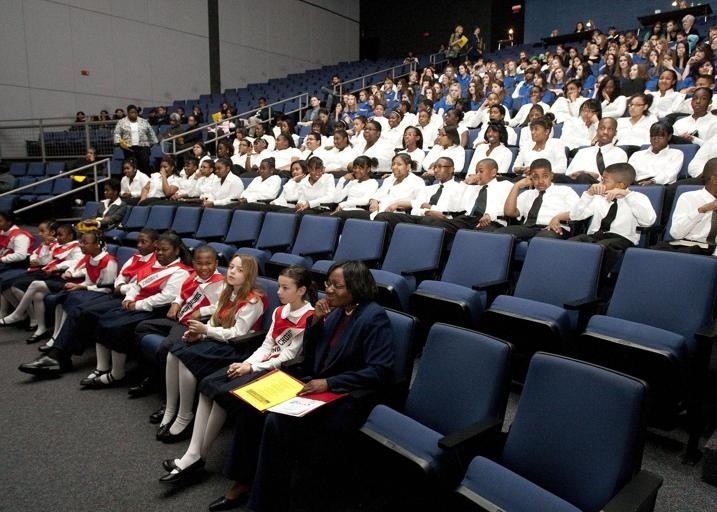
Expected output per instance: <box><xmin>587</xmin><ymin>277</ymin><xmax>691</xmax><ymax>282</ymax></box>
<box><xmin>92</xmin><ymin>371</ymin><xmax>129</xmax><ymax>389</ymax></box>
<box><xmin>80</xmin><ymin>369</ymin><xmax>111</xmax><ymax>386</ymax></box>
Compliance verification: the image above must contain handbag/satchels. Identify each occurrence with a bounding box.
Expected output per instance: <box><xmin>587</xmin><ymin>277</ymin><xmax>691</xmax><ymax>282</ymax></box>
<box><xmin>163</xmin><ymin>130</ymin><xmax>185</xmax><ymax>145</ymax></box>
<box><xmin>76</xmin><ymin>218</ymin><xmax>102</xmax><ymax>237</ymax></box>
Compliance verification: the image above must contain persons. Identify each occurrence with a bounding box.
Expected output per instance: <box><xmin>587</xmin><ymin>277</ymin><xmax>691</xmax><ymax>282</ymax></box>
<box><xmin>0</xmin><ymin>163</ymin><xmax>17</xmax><ymax>194</ymax></box>
<box><xmin>70</xmin><ymin>1</ymin><xmax>717</xmax><ymax>265</ymax></box>
<box><xmin>1</xmin><ymin>212</ymin><xmax>401</xmax><ymax>512</ymax></box>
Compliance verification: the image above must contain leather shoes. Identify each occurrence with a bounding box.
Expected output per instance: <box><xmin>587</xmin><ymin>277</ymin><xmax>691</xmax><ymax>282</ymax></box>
<box><xmin>127</xmin><ymin>377</ymin><xmax>162</xmax><ymax>396</ymax></box>
<box><xmin>18</xmin><ymin>355</ymin><xmax>61</xmax><ymax>375</ymax></box>
<box><xmin>148</xmin><ymin>400</ymin><xmax>195</xmax><ymax>445</ymax></box>
<box><xmin>163</xmin><ymin>459</ymin><xmax>179</xmax><ymax>473</ymax></box>
<box><xmin>27</xmin><ymin>330</ymin><xmax>50</xmax><ymax>344</ymax></box>
<box><xmin>39</xmin><ymin>337</ymin><xmax>56</xmax><ymax>353</ymax></box>
<box><xmin>208</xmin><ymin>496</ymin><xmax>248</xmax><ymax>512</ymax></box>
<box><xmin>159</xmin><ymin>457</ymin><xmax>204</xmax><ymax>484</ymax></box>
<box><xmin>0</xmin><ymin>315</ymin><xmax>37</xmax><ymax>332</ymax></box>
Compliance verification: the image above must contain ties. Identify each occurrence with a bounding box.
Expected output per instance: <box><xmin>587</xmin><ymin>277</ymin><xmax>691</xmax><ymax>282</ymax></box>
<box><xmin>706</xmin><ymin>208</ymin><xmax>717</xmax><ymax>255</ymax></box>
<box><xmin>592</xmin><ymin>198</ymin><xmax>616</xmax><ymax>242</ymax></box>
<box><xmin>596</xmin><ymin>148</ymin><xmax>605</xmax><ymax>177</ymax></box>
<box><xmin>522</xmin><ymin>191</ymin><xmax>546</xmax><ymax>229</ymax></box>
<box><xmin>466</xmin><ymin>185</ymin><xmax>488</xmax><ymax>221</ymax></box>
<box><xmin>429</xmin><ymin>185</ymin><xmax>444</xmax><ymax>205</ymax></box>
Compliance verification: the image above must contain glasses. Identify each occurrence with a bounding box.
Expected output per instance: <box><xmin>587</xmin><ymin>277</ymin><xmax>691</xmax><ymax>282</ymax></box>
<box><xmin>433</xmin><ymin>165</ymin><xmax>451</xmax><ymax>168</ymax></box>
<box><xmin>323</xmin><ymin>280</ymin><xmax>347</xmax><ymax>289</ymax></box>
<box><xmin>362</xmin><ymin>127</ymin><xmax>376</xmax><ymax>130</ymax></box>
<box><xmin>627</xmin><ymin>103</ymin><xmax>645</xmax><ymax>107</ymax></box>
<box><xmin>79</xmin><ymin>239</ymin><xmax>95</xmax><ymax>245</ymax></box>
